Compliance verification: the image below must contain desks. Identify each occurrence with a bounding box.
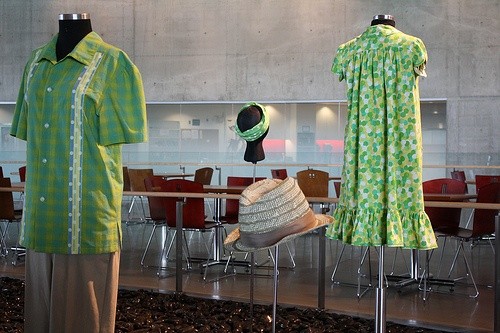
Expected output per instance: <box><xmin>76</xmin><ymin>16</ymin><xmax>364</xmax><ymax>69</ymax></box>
<box><xmin>114</xmin><ymin>176</ymin><xmax>478</xmax><ymax>247</ymax></box>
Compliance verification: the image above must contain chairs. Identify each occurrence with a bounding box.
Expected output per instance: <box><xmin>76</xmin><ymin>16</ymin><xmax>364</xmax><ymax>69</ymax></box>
<box><xmin>0</xmin><ymin>166</ymin><xmax>27</xmax><ymax>266</ymax></box>
<box><xmin>122</xmin><ymin>167</ymin><xmax>500</xmax><ymax>305</ymax></box>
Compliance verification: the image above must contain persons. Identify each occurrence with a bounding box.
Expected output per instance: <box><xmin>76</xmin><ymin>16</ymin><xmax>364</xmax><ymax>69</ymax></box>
<box><xmin>324</xmin><ymin>14</ymin><xmax>438</xmax><ymax>252</ymax></box>
<box><xmin>11</xmin><ymin>13</ymin><xmax>147</xmax><ymax>333</ymax></box>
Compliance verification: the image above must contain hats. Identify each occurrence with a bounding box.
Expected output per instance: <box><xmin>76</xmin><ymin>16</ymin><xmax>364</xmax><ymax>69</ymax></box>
<box><xmin>223</xmin><ymin>176</ymin><xmax>335</xmax><ymax>253</ymax></box>
